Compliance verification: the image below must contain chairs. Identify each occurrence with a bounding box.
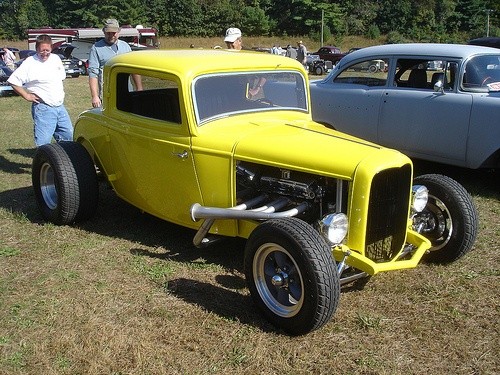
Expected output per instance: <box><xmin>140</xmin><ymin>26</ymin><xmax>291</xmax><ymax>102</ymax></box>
<box><xmin>404</xmin><ymin>69</ymin><xmax>450</xmax><ymax>88</ymax></box>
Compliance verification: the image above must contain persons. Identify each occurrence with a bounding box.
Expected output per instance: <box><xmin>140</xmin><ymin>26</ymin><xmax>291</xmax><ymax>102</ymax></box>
<box><xmin>87</xmin><ymin>18</ymin><xmax>146</xmax><ymax>109</ymax></box>
<box><xmin>7</xmin><ymin>35</ymin><xmax>74</xmax><ymax>149</ymax></box>
<box><xmin>213</xmin><ymin>27</ymin><xmax>308</xmax><ymax>101</ymax></box>
<box><xmin>2</xmin><ymin>44</ymin><xmax>17</xmax><ymax>94</ymax></box>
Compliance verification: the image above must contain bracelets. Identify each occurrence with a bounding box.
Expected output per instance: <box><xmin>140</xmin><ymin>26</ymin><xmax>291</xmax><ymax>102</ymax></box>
<box><xmin>257</xmin><ymin>84</ymin><xmax>261</xmax><ymax>87</ymax></box>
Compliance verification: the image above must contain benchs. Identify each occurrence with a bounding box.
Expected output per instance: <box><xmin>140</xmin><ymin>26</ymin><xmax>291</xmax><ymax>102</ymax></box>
<box><xmin>135</xmin><ymin>86</ymin><xmax>238</xmax><ymax>124</ymax></box>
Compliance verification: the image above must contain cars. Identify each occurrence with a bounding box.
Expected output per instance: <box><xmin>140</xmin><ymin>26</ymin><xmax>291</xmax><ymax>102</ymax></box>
<box><xmin>31</xmin><ymin>48</ymin><xmax>479</xmax><ymax>335</ymax></box>
<box><xmin>305</xmin><ymin>44</ymin><xmax>386</xmax><ymax>75</ymax></box>
<box><xmin>467</xmin><ymin>36</ymin><xmax>500</xmax><ymax>50</ymax></box>
<box><xmin>307</xmin><ymin>40</ymin><xmax>499</xmax><ymax>174</ymax></box>
<box><xmin>22</xmin><ymin>43</ymin><xmax>82</xmax><ymax>80</ymax></box>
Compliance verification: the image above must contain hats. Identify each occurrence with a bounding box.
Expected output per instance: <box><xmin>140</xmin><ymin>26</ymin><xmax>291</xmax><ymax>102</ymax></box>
<box><xmin>224</xmin><ymin>28</ymin><xmax>241</xmax><ymax>42</ymax></box>
<box><xmin>296</xmin><ymin>41</ymin><xmax>303</xmax><ymax>44</ymax></box>
<box><xmin>102</xmin><ymin>18</ymin><xmax>121</xmax><ymax>33</ymax></box>
